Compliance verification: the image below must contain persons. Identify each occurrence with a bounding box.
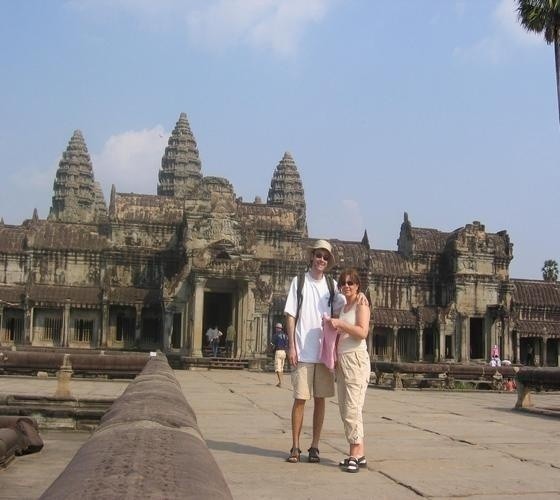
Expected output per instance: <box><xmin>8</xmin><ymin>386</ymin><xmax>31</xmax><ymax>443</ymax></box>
<box><xmin>273</xmin><ymin>323</ymin><xmax>287</xmax><ymax>388</ymax></box>
<box><xmin>515</xmin><ymin>380</ymin><xmax>535</xmax><ymax>409</ymax></box>
<box><xmin>490</xmin><ymin>344</ymin><xmax>502</xmax><ymax>367</ymax></box>
<box><xmin>283</xmin><ymin>239</ymin><xmax>371</xmax><ymax>472</ymax></box>
<box><xmin>503</xmin><ymin>377</ymin><xmax>517</xmax><ymax>392</ymax></box>
<box><xmin>205</xmin><ymin>325</ymin><xmax>223</xmax><ymax>356</ymax></box>
<box><xmin>524</xmin><ymin>343</ymin><xmax>534</xmax><ymax>366</ymax></box>
<box><xmin>225</xmin><ymin>322</ymin><xmax>237</xmax><ymax>358</ymax></box>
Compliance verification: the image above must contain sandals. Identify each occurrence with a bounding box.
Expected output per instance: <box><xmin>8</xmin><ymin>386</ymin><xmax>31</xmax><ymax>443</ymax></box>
<box><xmin>308</xmin><ymin>448</ymin><xmax>320</xmax><ymax>462</ymax></box>
<box><xmin>340</xmin><ymin>456</ymin><xmax>367</xmax><ymax>473</ymax></box>
<box><xmin>288</xmin><ymin>448</ymin><xmax>300</xmax><ymax>463</ymax></box>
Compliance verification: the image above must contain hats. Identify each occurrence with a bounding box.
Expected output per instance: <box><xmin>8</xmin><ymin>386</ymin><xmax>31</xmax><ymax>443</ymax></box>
<box><xmin>309</xmin><ymin>240</ymin><xmax>334</xmax><ymax>259</ymax></box>
<box><xmin>275</xmin><ymin>323</ymin><xmax>283</xmax><ymax>329</ymax></box>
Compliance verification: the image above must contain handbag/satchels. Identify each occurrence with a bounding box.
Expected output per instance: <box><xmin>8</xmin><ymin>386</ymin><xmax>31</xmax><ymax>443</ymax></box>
<box><xmin>319</xmin><ymin>313</ymin><xmax>338</xmax><ymax>371</ymax></box>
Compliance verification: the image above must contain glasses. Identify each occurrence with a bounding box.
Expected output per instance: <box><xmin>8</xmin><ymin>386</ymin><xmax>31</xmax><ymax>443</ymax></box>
<box><xmin>313</xmin><ymin>253</ymin><xmax>330</xmax><ymax>260</ymax></box>
<box><xmin>341</xmin><ymin>281</ymin><xmax>354</xmax><ymax>285</ymax></box>
